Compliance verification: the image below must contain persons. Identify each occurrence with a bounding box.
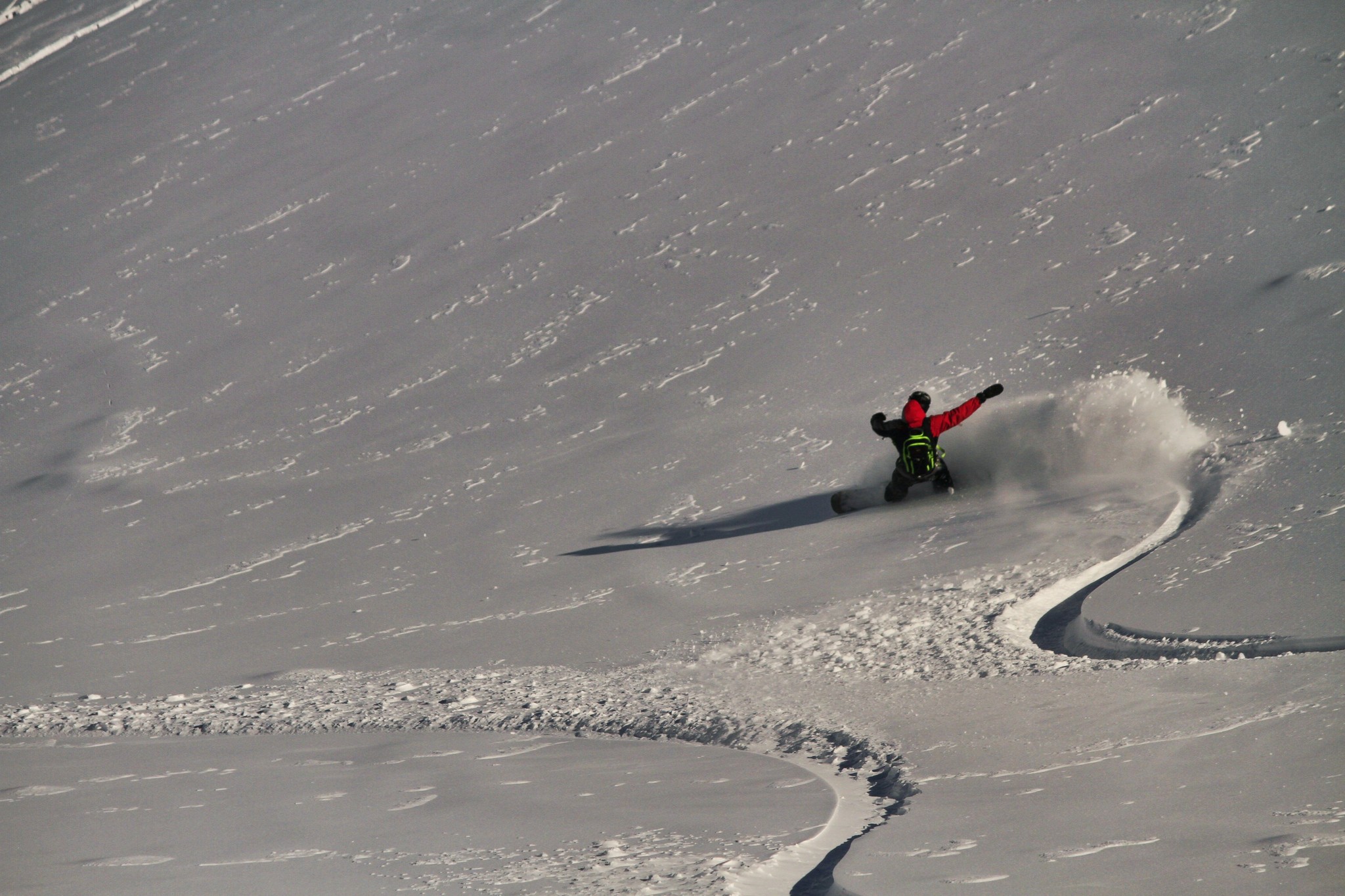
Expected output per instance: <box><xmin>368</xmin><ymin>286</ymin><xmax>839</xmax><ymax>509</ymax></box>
<box><xmin>870</xmin><ymin>384</ymin><xmax>1004</xmax><ymax>503</ymax></box>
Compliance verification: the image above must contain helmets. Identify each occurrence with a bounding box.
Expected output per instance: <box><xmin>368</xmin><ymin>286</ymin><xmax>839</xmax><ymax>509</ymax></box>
<box><xmin>907</xmin><ymin>391</ymin><xmax>930</xmax><ymax>407</ymax></box>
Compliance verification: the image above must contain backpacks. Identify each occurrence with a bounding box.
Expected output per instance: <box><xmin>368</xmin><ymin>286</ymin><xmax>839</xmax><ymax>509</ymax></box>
<box><xmin>897</xmin><ymin>419</ymin><xmax>936</xmax><ymax>476</ymax></box>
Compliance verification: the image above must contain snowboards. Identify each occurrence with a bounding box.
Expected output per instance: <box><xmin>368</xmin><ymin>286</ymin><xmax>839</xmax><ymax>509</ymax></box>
<box><xmin>830</xmin><ymin>456</ymin><xmax>994</xmax><ymax>514</ymax></box>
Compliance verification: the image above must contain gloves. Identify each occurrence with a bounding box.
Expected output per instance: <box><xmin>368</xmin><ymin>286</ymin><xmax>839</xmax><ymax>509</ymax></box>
<box><xmin>976</xmin><ymin>383</ymin><xmax>1003</xmax><ymax>403</ymax></box>
<box><xmin>873</xmin><ymin>412</ymin><xmax>886</xmax><ymax>420</ymax></box>
